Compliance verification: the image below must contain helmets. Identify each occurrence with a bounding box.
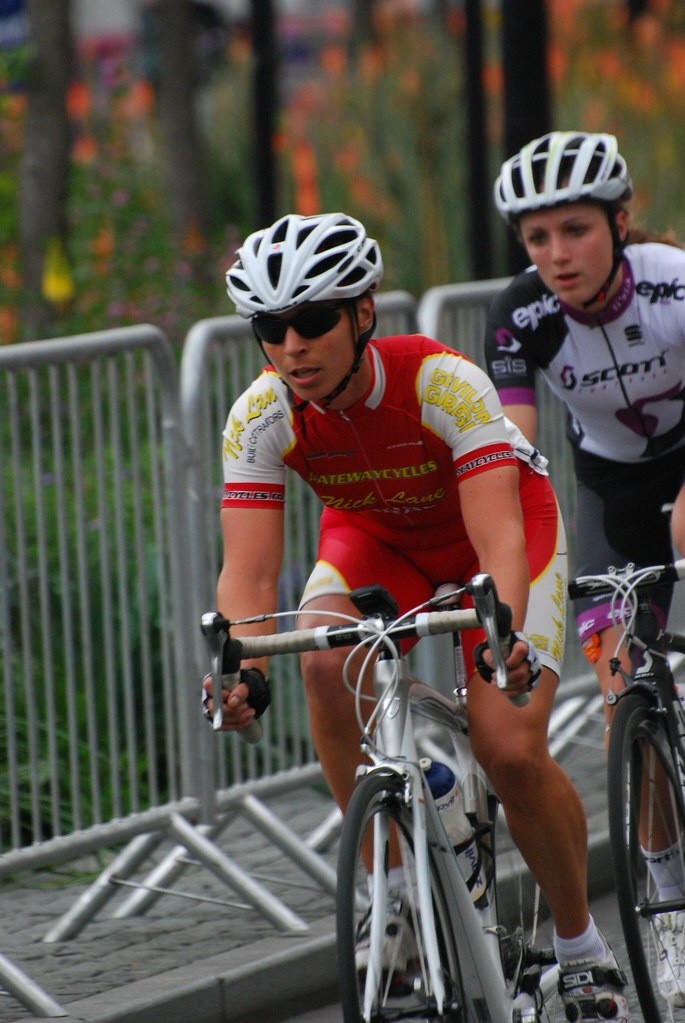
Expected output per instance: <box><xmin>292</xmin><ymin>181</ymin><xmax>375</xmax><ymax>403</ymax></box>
<box><xmin>225</xmin><ymin>213</ymin><xmax>383</xmax><ymax>320</ymax></box>
<box><xmin>491</xmin><ymin>130</ymin><xmax>633</xmax><ymax>229</ymax></box>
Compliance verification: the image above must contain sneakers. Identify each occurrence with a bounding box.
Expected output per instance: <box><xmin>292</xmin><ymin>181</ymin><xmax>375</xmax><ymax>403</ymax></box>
<box><xmin>552</xmin><ymin>928</ymin><xmax>628</xmax><ymax>1023</ymax></box>
<box><xmin>354</xmin><ymin>903</ymin><xmax>407</xmax><ymax>982</ymax></box>
<box><xmin>649</xmin><ymin>912</ymin><xmax>685</xmax><ymax>1007</ymax></box>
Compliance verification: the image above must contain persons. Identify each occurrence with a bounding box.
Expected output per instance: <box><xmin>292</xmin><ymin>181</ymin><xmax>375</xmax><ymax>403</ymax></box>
<box><xmin>485</xmin><ymin>130</ymin><xmax>685</xmax><ymax>1008</ymax></box>
<box><xmin>201</xmin><ymin>210</ymin><xmax>632</xmax><ymax>1023</ymax></box>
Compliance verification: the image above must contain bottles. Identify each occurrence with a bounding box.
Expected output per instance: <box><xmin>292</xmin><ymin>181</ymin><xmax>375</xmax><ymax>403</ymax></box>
<box><xmin>420</xmin><ymin>758</ymin><xmax>488</xmax><ymax>903</ymax></box>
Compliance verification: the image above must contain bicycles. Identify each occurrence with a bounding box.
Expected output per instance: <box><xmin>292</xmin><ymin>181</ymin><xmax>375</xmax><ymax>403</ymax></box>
<box><xmin>554</xmin><ymin>503</ymin><xmax>685</xmax><ymax>1022</ymax></box>
<box><xmin>199</xmin><ymin>570</ymin><xmax>561</xmax><ymax>1023</ymax></box>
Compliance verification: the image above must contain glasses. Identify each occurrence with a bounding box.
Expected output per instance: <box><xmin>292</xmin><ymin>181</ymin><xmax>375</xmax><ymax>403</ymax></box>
<box><xmin>249</xmin><ymin>306</ymin><xmax>346</xmax><ymax>345</ymax></box>
